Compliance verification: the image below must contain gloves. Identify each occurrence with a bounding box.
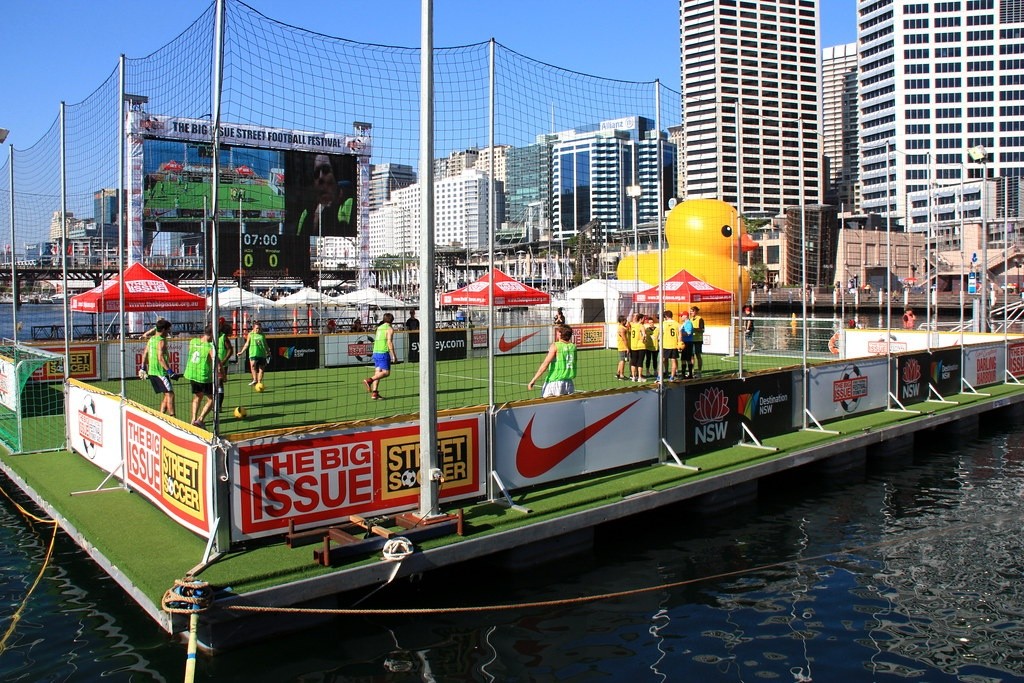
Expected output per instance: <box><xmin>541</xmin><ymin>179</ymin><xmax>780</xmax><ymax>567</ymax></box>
<box><xmin>170</xmin><ymin>372</ymin><xmax>179</xmax><ymax>381</ymax></box>
<box><xmin>139</xmin><ymin>368</ymin><xmax>148</xmax><ymax>380</ymax></box>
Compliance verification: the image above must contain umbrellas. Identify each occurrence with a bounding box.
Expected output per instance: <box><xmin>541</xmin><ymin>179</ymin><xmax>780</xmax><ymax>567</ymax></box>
<box><xmin>206</xmin><ymin>286</ymin><xmax>409</xmax><ymax>309</ymax></box>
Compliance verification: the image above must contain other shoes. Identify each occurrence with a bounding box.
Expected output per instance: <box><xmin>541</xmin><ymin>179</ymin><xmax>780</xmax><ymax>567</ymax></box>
<box><xmin>749</xmin><ymin>344</ymin><xmax>755</xmax><ymax>351</ymax></box>
<box><xmin>745</xmin><ymin>350</ymin><xmax>751</xmax><ymax>352</ymax></box>
<box><xmin>249</xmin><ymin>381</ymin><xmax>257</xmax><ymax>386</ymax></box>
<box><xmin>193</xmin><ymin>420</ymin><xmax>206</xmax><ymax>430</ymax></box>
<box><xmin>615</xmin><ymin>373</ymin><xmax>701</xmax><ymax>384</ymax></box>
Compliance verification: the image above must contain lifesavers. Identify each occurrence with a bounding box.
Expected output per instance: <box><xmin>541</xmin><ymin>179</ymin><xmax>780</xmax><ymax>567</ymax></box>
<box><xmin>827</xmin><ymin>332</ymin><xmax>840</xmax><ymax>356</ymax></box>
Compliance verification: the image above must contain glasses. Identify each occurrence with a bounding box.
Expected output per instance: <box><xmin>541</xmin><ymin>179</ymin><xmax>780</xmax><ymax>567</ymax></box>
<box><xmin>313</xmin><ymin>165</ymin><xmax>334</xmax><ymax>178</ymax></box>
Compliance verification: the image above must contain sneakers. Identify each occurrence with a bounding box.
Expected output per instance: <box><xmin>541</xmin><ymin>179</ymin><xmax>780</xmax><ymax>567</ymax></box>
<box><xmin>363</xmin><ymin>378</ymin><xmax>372</xmax><ymax>392</ymax></box>
<box><xmin>371</xmin><ymin>394</ymin><xmax>384</xmax><ymax>400</ymax></box>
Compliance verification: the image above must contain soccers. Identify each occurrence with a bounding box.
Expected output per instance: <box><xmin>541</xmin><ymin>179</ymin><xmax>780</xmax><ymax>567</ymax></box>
<box><xmin>255</xmin><ymin>382</ymin><xmax>265</xmax><ymax>393</ymax></box>
<box><xmin>234</xmin><ymin>407</ymin><xmax>248</xmax><ymax>419</ymax></box>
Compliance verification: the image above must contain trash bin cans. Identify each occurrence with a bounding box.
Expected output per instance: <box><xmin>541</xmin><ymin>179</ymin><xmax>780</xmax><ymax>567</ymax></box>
<box><xmin>776</xmin><ymin>324</ymin><xmax>791</xmax><ymax>350</ymax></box>
<box><xmin>864</xmin><ymin>287</ymin><xmax>870</xmax><ymax>294</ymax></box>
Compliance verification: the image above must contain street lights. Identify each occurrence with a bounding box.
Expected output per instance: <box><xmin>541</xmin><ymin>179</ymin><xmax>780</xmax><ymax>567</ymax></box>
<box><xmin>910</xmin><ymin>264</ymin><xmax>917</xmax><ymax>286</ymax></box>
<box><xmin>1014</xmin><ymin>261</ymin><xmax>1022</xmax><ymax>295</ymax></box>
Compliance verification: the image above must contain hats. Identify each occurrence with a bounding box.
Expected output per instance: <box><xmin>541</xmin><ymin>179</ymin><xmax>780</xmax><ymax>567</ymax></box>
<box><xmin>678</xmin><ymin>311</ymin><xmax>689</xmax><ymax>316</ymax></box>
<box><xmin>744</xmin><ymin>307</ymin><xmax>750</xmax><ymax>311</ymax></box>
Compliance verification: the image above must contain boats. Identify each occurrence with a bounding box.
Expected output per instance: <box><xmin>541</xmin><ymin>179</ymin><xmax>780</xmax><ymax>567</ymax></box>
<box><xmin>0</xmin><ymin>283</ymin><xmax>78</xmax><ymax>304</ymax></box>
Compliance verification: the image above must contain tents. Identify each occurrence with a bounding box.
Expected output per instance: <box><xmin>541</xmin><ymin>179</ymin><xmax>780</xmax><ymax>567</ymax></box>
<box><xmin>236</xmin><ymin>165</ymin><xmax>254</xmax><ymax>184</ymax></box>
<box><xmin>632</xmin><ymin>269</ymin><xmax>734</xmax><ymax>319</ymax></box>
<box><xmin>440</xmin><ymin>268</ymin><xmax>551</xmax><ymax>329</ymax></box>
<box><xmin>163</xmin><ymin>160</ymin><xmax>183</xmax><ymax>182</ymax></box>
<box><xmin>565</xmin><ymin>279</ymin><xmax>659</xmax><ymax>324</ymax></box>
<box><xmin>70</xmin><ymin>263</ymin><xmax>207</xmax><ymax>343</ymax></box>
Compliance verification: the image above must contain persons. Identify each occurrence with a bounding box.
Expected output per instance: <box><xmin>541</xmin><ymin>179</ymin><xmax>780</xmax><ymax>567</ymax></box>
<box><xmin>220</xmin><ymin>317</ymin><xmax>232</xmax><ymax>334</ymax></box>
<box><xmin>405</xmin><ymin>309</ymin><xmax>419</xmax><ymax>330</ymax></box>
<box><xmin>364</xmin><ymin>313</ymin><xmax>397</xmax><ymax>400</ymax></box>
<box><xmin>615</xmin><ymin>306</ymin><xmax>704</xmax><ymax>383</ymax></box>
<box><xmin>231</xmin><ymin>187</ymin><xmax>246</xmax><ymax>202</ymax></box>
<box><xmin>528</xmin><ymin>325</ymin><xmax>578</xmax><ymax>398</ymax></box>
<box><xmin>138</xmin><ymin>316</ymin><xmax>234</xmax><ymax>431</ymax></box>
<box><xmin>237</xmin><ymin>322</ymin><xmax>271</xmax><ymax>386</ymax></box>
<box><xmin>745</xmin><ymin>308</ymin><xmax>756</xmax><ymax>351</ymax></box>
<box><xmin>297</xmin><ymin>155</ymin><xmax>357</xmax><ymax>237</ymax></box>
<box><xmin>324</xmin><ymin>319</ymin><xmax>336</xmax><ymax>333</ymax></box>
<box><xmin>903</xmin><ymin>307</ymin><xmax>917</xmax><ymax>330</ymax></box>
<box><xmin>349</xmin><ymin>319</ymin><xmax>365</xmax><ymax>332</ymax></box>
<box><xmin>892</xmin><ymin>290</ymin><xmax>897</xmax><ymax>301</ymax></box>
<box><xmin>554</xmin><ymin>308</ymin><xmax>565</xmax><ymax>324</ymax></box>
<box><xmin>148</xmin><ymin>173</ymin><xmax>188</xmax><ymax>208</ymax></box>
